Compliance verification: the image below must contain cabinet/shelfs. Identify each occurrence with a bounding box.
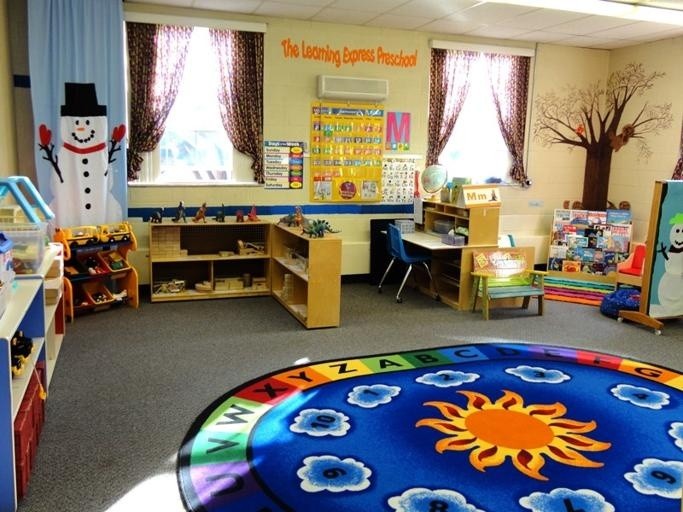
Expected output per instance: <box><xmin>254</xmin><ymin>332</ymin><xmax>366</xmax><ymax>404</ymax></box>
<box><xmin>1</xmin><ymin>240</ymin><xmax>67</xmax><ymax>511</ymax></box>
<box><xmin>148</xmin><ymin>217</ymin><xmax>342</xmax><ymax>332</ymax></box>
<box><xmin>411</xmin><ymin>199</ymin><xmax>500</xmax><ymax>312</ymax></box>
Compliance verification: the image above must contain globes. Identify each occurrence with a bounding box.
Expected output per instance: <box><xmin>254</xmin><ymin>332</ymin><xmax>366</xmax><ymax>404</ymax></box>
<box><xmin>421</xmin><ymin>164</ymin><xmax>449</xmax><ymax>201</ymax></box>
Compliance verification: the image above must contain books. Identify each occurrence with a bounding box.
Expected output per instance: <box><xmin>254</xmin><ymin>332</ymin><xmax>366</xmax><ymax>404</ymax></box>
<box><xmin>546</xmin><ymin>208</ymin><xmax>633</xmax><ymax>276</ymax></box>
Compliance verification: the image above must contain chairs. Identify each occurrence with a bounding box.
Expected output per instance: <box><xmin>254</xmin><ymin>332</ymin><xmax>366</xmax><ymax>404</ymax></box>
<box><xmin>377</xmin><ymin>224</ymin><xmax>441</xmax><ymax>303</ymax></box>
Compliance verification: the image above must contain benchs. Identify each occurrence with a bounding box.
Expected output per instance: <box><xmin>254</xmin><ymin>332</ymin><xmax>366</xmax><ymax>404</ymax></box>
<box><xmin>468</xmin><ymin>246</ymin><xmax>550</xmax><ymax>321</ymax></box>
